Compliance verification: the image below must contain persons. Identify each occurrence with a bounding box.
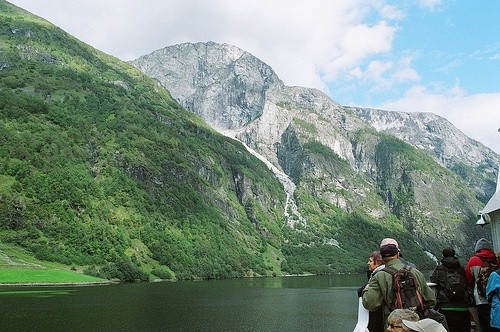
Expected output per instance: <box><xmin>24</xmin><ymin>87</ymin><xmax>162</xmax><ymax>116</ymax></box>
<box><xmin>402</xmin><ymin>318</ymin><xmax>447</xmax><ymax>332</ymax></box>
<box><xmin>466</xmin><ymin>238</ymin><xmax>498</xmax><ymax>332</ymax></box>
<box><xmin>387</xmin><ymin>309</ymin><xmax>419</xmax><ymax>332</ymax></box>
<box><xmin>486</xmin><ymin>249</ymin><xmax>500</xmax><ymax>332</ymax></box>
<box><xmin>358</xmin><ymin>252</ymin><xmax>384</xmax><ymax>332</ymax></box>
<box><xmin>430</xmin><ymin>247</ymin><xmax>472</xmax><ymax>332</ymax></box>
<box><xmin>362</xmin><ymin>238</ymin><xmax>436</xmax><ymax>319</ymax></box>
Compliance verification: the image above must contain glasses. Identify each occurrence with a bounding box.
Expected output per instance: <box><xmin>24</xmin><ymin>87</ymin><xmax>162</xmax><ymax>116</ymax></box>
<box><xmin>387</xmin><ymin>323</ymin><xmax>402</xmax><ymax>328</ymax></box>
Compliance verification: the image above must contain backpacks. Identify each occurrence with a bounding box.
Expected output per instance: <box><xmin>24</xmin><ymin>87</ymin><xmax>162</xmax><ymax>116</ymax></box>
<box><xmin>379</xmin><ymin>264</ymin><xmax>426</xmax><ymax>320</ymax></box>
<box><xmin>435</xmin><ymin>264</ymin><xmax>466</xmax><ymax>309</ymax></box>
<box><xmin>475</xmin><ymin>255</ymin><xmax>499</xmax><ymax>298</ymax></box>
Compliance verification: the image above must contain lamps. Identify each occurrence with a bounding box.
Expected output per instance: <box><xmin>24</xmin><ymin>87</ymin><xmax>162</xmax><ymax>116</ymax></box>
<box><xmin>475</xmin><ymin>215</ymin><xmax>490</xmax><ymax>229</ymax></box>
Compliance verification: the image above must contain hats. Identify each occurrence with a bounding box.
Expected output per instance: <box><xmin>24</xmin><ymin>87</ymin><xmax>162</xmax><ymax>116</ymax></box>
<box><xmin>380</xmin><ymin>243</ymin><xmax>401</xmax><ymax>258</ymax></box>
<box><xmin>380</xmin><ymin>237</ymin><xmax>401</xmax><ymax>251</ymax></box>
<box><xmin>475</xmin><ymin>237</ymin><xmax>492</xmax><ymax>254</ymax></box>
<box><xmin>401</xmin><ymin>318</ymin><xmax>448</xmax><ymax>332</ymax></box>
